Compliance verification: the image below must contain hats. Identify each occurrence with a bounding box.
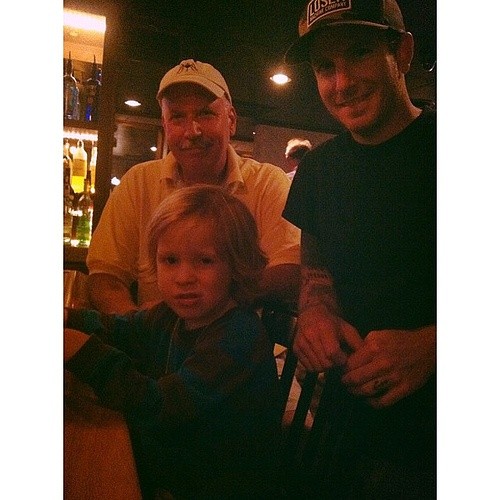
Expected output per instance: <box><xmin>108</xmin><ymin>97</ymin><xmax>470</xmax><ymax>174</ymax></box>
<box><xmin>156</xmin><ymin>59</ymin><xmax>232</xmax><ymax>105</ymax></box>
<box><xmin>283</xmin><ymin>0</ymin><xmax>407</xmax><ymax>67</ymax></box>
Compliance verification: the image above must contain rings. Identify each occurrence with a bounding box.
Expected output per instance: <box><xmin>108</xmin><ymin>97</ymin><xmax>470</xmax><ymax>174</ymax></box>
<box><xmin>373</xmin><ymin>379</ymin><xmax>388</xmax><ymax>392</ymax></box>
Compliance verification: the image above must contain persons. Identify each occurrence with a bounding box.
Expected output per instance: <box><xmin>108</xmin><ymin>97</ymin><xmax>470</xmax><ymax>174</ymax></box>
<box><xmin>84</xmin><ymin>60</ymin><xmax>315</xmax><ymax>429</ymax></box>
<box><xmin>283</xmin><ymin>136</ymin><xmax>312</xmax><ymax>183</ymax></box>
<box><xmin>63</xmin><ymin>185</ymin><xmax>287</xmax><ymax>500</ymax></box>
<box><xmin>278</xmin><ymin>0</ymin><xmax>438</xmax><ymax>500</ymax></box>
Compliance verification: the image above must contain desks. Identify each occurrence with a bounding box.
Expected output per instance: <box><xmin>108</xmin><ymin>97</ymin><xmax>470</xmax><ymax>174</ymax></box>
<box><xmin>65</xmin><ymin>328</ymin><xmax>142</xmax><ymax>500</ymax></box>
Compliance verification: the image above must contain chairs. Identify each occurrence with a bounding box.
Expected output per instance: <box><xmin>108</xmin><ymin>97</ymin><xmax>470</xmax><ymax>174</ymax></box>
<box><xmin>260</xmin><ymin>301</ymin><xmax>350</xmax><ymax>465</ymax></box>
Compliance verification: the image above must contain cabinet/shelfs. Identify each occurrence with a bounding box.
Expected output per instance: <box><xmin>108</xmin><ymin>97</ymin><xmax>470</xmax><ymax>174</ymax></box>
<box><xmin>63</xmin><ymin>0</ymin><xmax>253</xmax><ymax>269</ymax></box>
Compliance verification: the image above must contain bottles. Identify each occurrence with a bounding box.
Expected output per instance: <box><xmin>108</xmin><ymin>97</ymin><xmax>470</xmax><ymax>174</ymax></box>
<box><xmin>63</xmin><ymin>58</ymin><xmax>101</xmax><ymax>249</ymax></box>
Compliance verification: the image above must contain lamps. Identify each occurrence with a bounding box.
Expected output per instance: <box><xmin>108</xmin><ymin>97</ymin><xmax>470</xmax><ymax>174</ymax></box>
<box><xmin>268</xmin><ymin>62</ymin><xmax>292</xmax><ymax>86</ymax></box>
